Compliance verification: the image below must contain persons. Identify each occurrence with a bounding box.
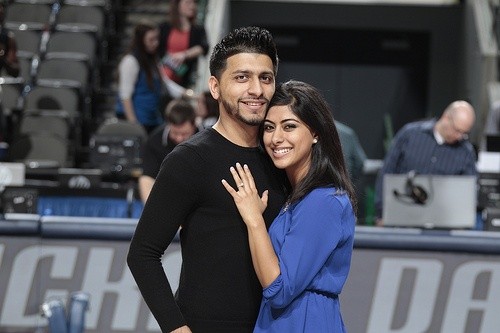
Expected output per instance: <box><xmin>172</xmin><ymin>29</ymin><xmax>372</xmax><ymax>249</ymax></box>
<box><xmin>1</xmin><ymin>0</ymin><xmax>367</xmax><ymax>224</ymax></box>
<box><xmin>222</xmin><ymin>80</ymin><xmax>356</xmax><ymax>333</ymax></box>
<box><xmin>375</xmin><ymin>100</ymin><xmax>477</xmax><ymax>225</ymax></box>
<box><xmin>126</xmin><ymin>26</ymin><xmax>292</xmax><ymax>333</ymax></box>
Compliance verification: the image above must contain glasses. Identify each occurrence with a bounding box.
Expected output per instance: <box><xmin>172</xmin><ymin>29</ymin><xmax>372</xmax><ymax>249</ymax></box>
<box><xmin>449</xmin><ymin>117</ymin><xmax>469</xmax><ymax>140</ymax></box>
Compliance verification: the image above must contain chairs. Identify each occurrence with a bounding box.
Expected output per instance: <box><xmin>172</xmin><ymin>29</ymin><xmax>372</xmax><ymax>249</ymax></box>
<box><xmin>0</xmin><ymin>0</ymin><xmax>205</xmax><ymax>162</ymax></box>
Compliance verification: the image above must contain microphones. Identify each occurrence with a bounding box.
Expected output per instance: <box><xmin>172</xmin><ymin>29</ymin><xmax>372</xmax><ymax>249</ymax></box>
<box><xmin>394</xmin><ymin>190</ymin><xmax>414</xmax><ymax>204</ymax></box>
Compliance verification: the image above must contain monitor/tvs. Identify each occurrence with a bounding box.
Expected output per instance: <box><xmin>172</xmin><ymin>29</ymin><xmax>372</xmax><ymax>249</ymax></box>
<box><xmin>382</xmin><ymin>174</ymin><xmax>478</xmax><ymax>231</ymax></box>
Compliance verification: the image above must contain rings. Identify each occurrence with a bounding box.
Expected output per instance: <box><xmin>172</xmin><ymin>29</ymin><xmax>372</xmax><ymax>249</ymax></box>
<box><xmin>237</xmin><ymin>183</ymin><xmax>244</xmax><ymax>188</ymax></box>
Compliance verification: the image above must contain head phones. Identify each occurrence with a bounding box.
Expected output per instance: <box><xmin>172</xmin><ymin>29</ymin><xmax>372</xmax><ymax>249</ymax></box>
<box><xmin>405</xmin><ymin>170</ymin><xmax>427</xmax><ymax>205</ymax></box>
